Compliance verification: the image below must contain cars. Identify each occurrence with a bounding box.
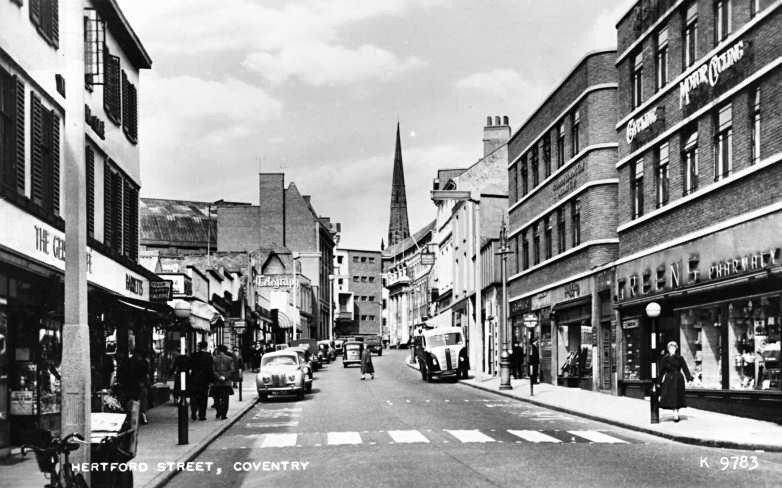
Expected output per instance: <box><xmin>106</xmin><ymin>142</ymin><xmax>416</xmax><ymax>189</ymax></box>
<box><xmin>416</xmin><ymin>326</ymin><xmax>470</xmax><ymax>384</ymax></box>
<box><xmin>255</xmin><ymin>335</ymin><xmax>382</xmax><ymax>401</ymax></box>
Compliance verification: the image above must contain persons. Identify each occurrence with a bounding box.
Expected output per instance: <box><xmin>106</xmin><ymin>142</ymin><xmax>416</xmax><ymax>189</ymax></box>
<box><xmin>361</xmin><ymin>343</ymin><xmax>374</xmax><ymax>379</ymax></box>
<box><xmin>127</xmin><ymin>340</ymin><xmax>275</xmax><ymax>424</ymax></box>
<box><xmin>512</xmin><ymin>338</ymin><xmax>540</xmax><ymax>384</ymax></box>
<box><xmin>656</xmin><ymin>341</ymin><xmax>694</xmax><ymax>422</ymax></box>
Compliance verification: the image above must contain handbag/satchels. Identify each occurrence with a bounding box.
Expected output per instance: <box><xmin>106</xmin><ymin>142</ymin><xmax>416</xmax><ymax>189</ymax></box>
<box><xmin>209</xmin><ymin>384</ymin><xmax>234</xmax><ymax>398</ymax></box>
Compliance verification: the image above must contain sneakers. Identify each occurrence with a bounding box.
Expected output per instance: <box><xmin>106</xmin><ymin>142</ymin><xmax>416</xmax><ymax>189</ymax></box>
<box><xmin>192</xmin><ymin>417</ymin><xmax>206</xmax><ymax>420</ymax></box>
<box><xmin>217</xmin><ymin>414</ymin><xmax>227</xmax><ymax>419</ymax></box>
<box><xmin>360</xmin><ymin>376</ymin><xmax>374</xmax><ymax>380</ymax></box>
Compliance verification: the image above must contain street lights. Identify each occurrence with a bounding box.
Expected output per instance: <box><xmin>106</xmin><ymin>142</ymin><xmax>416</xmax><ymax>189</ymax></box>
<box><xmin>644</xmin><ymin>301</ymin><xmax>662</xmax><ymax>424</ymax></box>
<box><xmin>292</xmin><ymin>251</ymin><xmax>301</xmax><ymax>346</ymax></box>
<box><xmin>327</xmin><ymin>274</ymin><xmax>334</xmax><ymax>341</ymax></box>
<box><xmin>494</xmin><ymin>213</ymin><xmax>514</xmax><ymax>389</ymax></box>
<box><xmin>206</xmin><ymin>199</ymin><xmax>225</xmax><ymax>267</ymax></box>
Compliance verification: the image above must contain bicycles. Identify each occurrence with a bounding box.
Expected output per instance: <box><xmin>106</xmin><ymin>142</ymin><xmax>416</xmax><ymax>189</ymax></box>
<box><xmin>19</xmin><ymin>419</ymin><xmax>139</xmax><ymax>487</ymax></box>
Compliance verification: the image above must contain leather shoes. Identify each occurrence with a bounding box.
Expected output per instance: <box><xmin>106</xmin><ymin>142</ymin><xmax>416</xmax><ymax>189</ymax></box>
<box><xmin>674</xmin><ymin>417</ymin><xmax>680</xmax><ymax>421</ymax></box>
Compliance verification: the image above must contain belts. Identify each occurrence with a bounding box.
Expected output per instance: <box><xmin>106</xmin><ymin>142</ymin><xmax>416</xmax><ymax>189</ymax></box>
<box><xmin>34</xmin><ymin>446</ymin><xmax>59</xmax><ymax>472</ymax></box>
